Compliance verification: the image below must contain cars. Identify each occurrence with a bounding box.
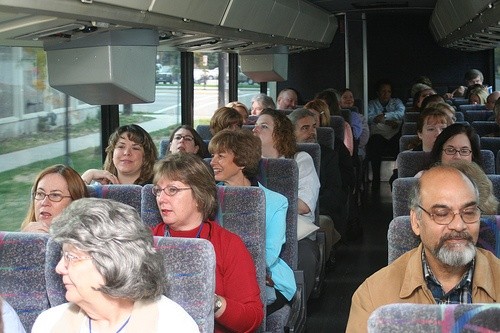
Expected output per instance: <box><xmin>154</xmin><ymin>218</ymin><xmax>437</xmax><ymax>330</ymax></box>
<box><xmin>154</xmin><ymin>62</ymin><xmax>259</xmax><ymax>85</ymax></box>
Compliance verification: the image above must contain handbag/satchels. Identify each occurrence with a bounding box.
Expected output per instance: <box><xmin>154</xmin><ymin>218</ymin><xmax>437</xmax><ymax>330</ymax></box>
<box><xmin>370</xmin><ymin>120</ymin><xmax>401</xmax><ymax>139</ymax></box>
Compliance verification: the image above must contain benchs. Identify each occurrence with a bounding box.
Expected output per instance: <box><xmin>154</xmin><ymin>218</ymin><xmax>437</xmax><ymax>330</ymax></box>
<box><xmin>0</xmin><ymin>98</ymin><xmax>363</xmax><ymax>333</ymax></box>
<box><xmin>367</xmin><ymin>95</ymin><xmax>500</xmax><ymax>333</ymax></box>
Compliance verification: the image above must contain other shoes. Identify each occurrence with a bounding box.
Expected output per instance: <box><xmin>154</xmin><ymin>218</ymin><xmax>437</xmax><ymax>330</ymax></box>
<box><xmin>373</xmin><ymin>170</ymin><xmax>381</xmax><ymax>181</ymax></box>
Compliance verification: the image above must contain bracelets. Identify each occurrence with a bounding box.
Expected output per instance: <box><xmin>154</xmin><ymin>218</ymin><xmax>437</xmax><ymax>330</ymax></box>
<box><xmin>214</xmin><ymin>293</ymin><xmax>223</xmax><ymax>314</ymax></box>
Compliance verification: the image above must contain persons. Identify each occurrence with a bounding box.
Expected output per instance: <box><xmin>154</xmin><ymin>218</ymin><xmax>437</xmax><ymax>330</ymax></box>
<box><xmin>209</xmin><ymin>69</ymin><xmax>500</xmax><ymax>216</ymax></box>
<box><xmin>276</xmin><ymin>88</ymin><xmax>298</xmax><ymax>111</ymax></box>
<box><xmin>167</xmin><ymin>125</ymin><xmax>212</xmax><ymax>158</ymax></box>
<box><xmin>31</xmin><ymin>198</ymin><xmax>201</xmax><ymax>333</ymax></box>
<box><xmin>366</xmin><ymin>80</ymin><xmax>406</xmax><ymax>193</ymax></box>
<box><xmin>80</xmin><ymin>124</ymin><xmax>159</xmax><ymax>187</ymax></box>
<box><xmin>386</xmin><ymin>108</ymin><xmax>454</xmax><ymax>193</ymax></box>
<box><xmin>344</xmin><ymin>166</ymin><xmax>500</xmax><ymax>333</ymax></box>
<box><xmin>411</xmin><ymin>123</ymin><xmax>481</xmax><ymax>178</ymax></box>
<box><xmin>288</xmin><ymin>107</ymin><xmax>317</xmax><ymax>143</ymax></box>
<box><xmin>149</xmin><ymin>151</ymin><xmax>264</xmax><ymax>333</ymax></box>
<box><xmin>20</xmin><ymin>163</ymin><xmax>90</xmax><ymax>234</ymax></box>
<box><xmin>208</xmin><ymin>126</ymin><xmax>297</xmax><ymax>316</ymax></box>
<box><xmin>0</xmin><ymin>296</ymin><xmax>28</xmax><ymax>333</ymax></box>
<box><xmin>251</xmin><ymin>108</ymin><xmax>322</xmax><ymax>304</ymax></box>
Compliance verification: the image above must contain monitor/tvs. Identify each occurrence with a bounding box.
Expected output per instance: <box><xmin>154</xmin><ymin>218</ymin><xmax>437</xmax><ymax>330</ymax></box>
<box><xmin>241</xmin><ymin>45</ymin><xmax>288</xmax><ymax>81</ymax></box>
<box><xmin>44</xmin><ymin>29</ymin><xmax>158</xmax><ymax>105</ymax></box>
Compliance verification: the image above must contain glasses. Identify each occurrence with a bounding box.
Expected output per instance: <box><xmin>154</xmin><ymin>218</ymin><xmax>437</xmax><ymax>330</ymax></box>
<box><xmin>173</xmin><ymin>135</ymin><xmax>194</xmax><ymax>141</ymax></box>
<box><xmin>61</xmin><ymin>251</ymin><xmax>94</xmax><ymax>268</ymax></box>
<box><xmin>151</xmin><ymin>185</ymin><xmax>192</xmax><ymax>197</ymax></box>
<box><xmin>417</xmin><ymin>204</ymin><xmax>484</xmax><ymax>225</ymax></box>
<box><xmin>442</xmin><ymin>148</ymin><xmax>472</xmax><ymax>156</ymax></box>
<box><xmin>32</xmin><ymin>192</ymin><xmax>72</xmax><ymax>202</ymax></box>
